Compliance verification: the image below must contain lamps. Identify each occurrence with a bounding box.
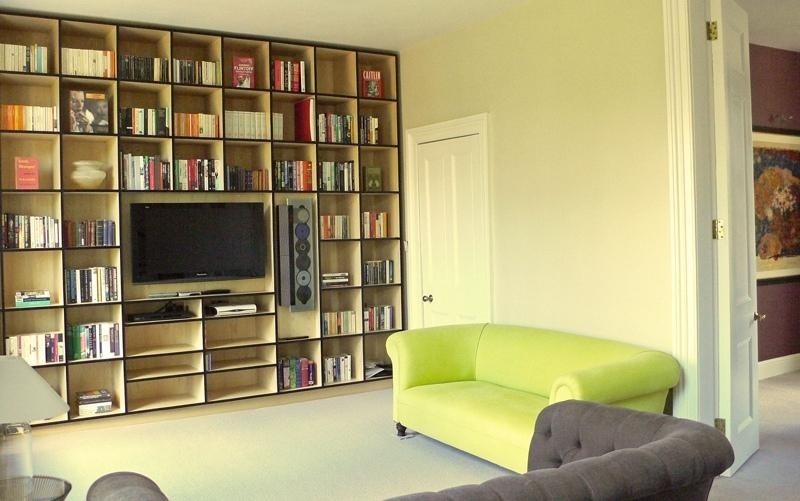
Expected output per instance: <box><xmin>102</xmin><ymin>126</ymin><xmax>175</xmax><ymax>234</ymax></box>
<box><xmin>1</xmin><ymin>356</ymin><xmax>71</xmax><ymax>495</ymax></box>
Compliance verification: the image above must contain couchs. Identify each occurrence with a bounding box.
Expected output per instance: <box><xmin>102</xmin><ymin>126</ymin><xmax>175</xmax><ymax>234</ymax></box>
<box><xmin>385</xmin><ymin>322</ymin><xmax>681</xmax><ymax>475</ymax></box>
<box><xmin>85</xmin><ymin>400</ymin><xmax>736</xmax><ymax>500</ymax></box>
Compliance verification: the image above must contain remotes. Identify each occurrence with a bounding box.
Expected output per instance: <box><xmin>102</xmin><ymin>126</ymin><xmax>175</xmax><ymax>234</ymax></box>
<box><xmin>148</xmin><ymin>292</ymin><xmax>177</xmax><ymax>297</ymax></box>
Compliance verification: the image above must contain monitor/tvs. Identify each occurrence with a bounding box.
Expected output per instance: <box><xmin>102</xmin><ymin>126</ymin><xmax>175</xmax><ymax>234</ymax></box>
<box><xmin>129</xmin><ymin>202</ymin><xmax>266</xmax><ymax>285</ymax></box>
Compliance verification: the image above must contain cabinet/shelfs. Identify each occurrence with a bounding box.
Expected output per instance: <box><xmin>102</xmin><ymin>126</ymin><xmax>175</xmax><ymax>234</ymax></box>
<box><xmin>1</xmin><ymin>12</ymin><xmax>404</xmax><ymax>430</ymax></box>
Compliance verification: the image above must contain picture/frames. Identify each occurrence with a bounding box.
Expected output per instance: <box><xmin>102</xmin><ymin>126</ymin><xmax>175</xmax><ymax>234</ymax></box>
<box><xmin>750</xmin><ymin>125</ymin><xmax>800</xmax><ymax>287</ymax></box>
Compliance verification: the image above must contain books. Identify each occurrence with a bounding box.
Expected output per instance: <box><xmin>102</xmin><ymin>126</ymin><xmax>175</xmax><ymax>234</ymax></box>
<box><xmin>0</xmin><ymin>104</ymin><xmax>58</xmax><ymax>133</ymax></box>
<box><xmin>171</xmin><ymin>58</ymin><xmax>216</xmax><ymax>86</ymax></box>
<box><xmin>60</xmin><ymin>46</ymin><xmax>115</xmax><ymax>79</ymax></box>
<box><xmin>363</xmin><ymin>260</ymin><xmax>394</xmax><ymax>284</ymax></box>
<box><xmin>361</xmin><ymin>211</ymin><xmax>387</xmax><ymax>239</ymax></box>
<box><xmin>294</xmin><ymin>98</ymin><xmax>315</xmax><ymax>142</ymax></box>
<box><xmin>320</xmin><ymin>215</ymin><xmax>350</xmax><ymax>239</ymax></box>
<box><xmin>120</xmin><ymin>151</ymin><xmax>170</xmax><ymax>191</ymax></box>
<box><xmin>14</xmin><ymin>157</ymin><xmax>39</xmax><ymax>189</ymax></box>
<box><xmin>271</xmin><ymin>55</ymin><xmax>306</xmax><ymax>94</ymax></box>
<box><xmin>62</xmin><ymin>220</ymin><xmax>116</xmax><ymax>247</ymax></box>
<box><xmin>64</xmin><ymin>265</ymin><xmax>118</xmax><ymax>304</ymax></box>
<box><xmin>364</xmin><ymin>166</ymin><xmax>382</xmax><ymax>193</ymax></box>
<box><xmin>66</xmin><ymin>321</ymin><xmax>120</xmax><ymax>359</ymax></box>
<box><xmin>322</xmin><ymin>272</ymin><xmax>349</xmax><ymax>288</ymax></box>
<box><xmin>224</xmin><ymin>163</ymin><xmax>269</xmax><ymax>191</ymax></box>
<box><xmin>271</xmin><ymin>112</ymin><xmax>283</xmax><ymax>141</ymax></box>
<box><xmin>68</xmin><ymin>90</ymin><xmax>109</xmax><ymax>134</ymax></box>
<box><xmin>0</xmin><ymin>42</ymin><xmax>48</xmax><ymax>75</ymax></box>
<box><xmin>319</xmin><ymin>113</ymin><xmax>354</xmax><ymax>144</ymax></box>
<box><xmin>205</xmin><ymin>352</ymin><xmax>211</xmax><ymax>372</ymax></box>
<box><xmin>359</xmin><ymin>115</ymin><xmax>379</xmax><ymax>145</ymax></box>
<box><xmin>75</xmin><ymin>388</ymin><xmax>113</xmax><ymax>416</ymax></box>
<box><xmin>361</xmin><ymin>68</ymin><xmax>384</xmax><ymax>98</ymax></box>
<box><xmin>317</xmin><ymin>160</ymin><xmax>355</xmax><ymax>192</ymax></box>
<box><xmin>0</xmin><ymin>213</ymin><xmax>59</xmax><ymax>248</ymax></box>
<box><xmin>322</xmin><ymin>310</ymin><xmax>357</xmax><ymax>336</ymax></box>
<box><xmin>278</xmin><ymin>356</ymin><xmax>315</xmax><ymax>390</ymax></box>
<box><xmin>231</xmin><ymin>55</ymin><xmax>256</xmax><ymax>90</ymax></box>
<box><xmin>118</xmin><ymin>107</ymin><xmax>170</xmax><ymax>137</ymax></box>
<box><xmin>4</xmin><ymin>331</ymin><xmax>64</xmax><ymax>365</ymax></box>
<box><xmin>323</xmin><ymin>353</ymin><xmax>352</xmax><ymax>385</ymax></box>
<box><xmin>15</xmin><ymin>288</ymin><xmax>51</xmax><ymax>307</ymax></box>
<box><xmin>174</xmin><ymin>159</ymin><xmax>222</xmax><ymax>190</ymax></box>
<box><xmin>117</xmin><ymin>53</ymin><xmax>168</xmax><ymax>84</ymax></box>
<box><xmin>173</xmin><ymin>112</ymin><xmax>219</xmax><ymax>138</ymax></box>
<box><xmin>272</xmin><ymin>160</ymin><xmax>316</xmax><ymax>191</ymax></box>
<box><xmin>363</xmin><ymin>303</ymin><xmax>393</xmax><ymax>331</ymax></box>
<box><xmin>224</xmin><ymin>109</ymin><xmax>267</xmax><ymax>140</ymax></box>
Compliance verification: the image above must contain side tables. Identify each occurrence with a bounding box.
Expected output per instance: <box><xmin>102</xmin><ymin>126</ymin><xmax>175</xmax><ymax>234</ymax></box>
<box><xmin>0</xmin><ymin>477</ymin><xmax>71</xmax><ymax>501</ymax></box>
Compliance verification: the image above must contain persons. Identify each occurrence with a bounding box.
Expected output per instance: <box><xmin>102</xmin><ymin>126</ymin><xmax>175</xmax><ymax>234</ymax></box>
<box><xmin>236</xmin><ymin>74</ymin><xmax>250</xmax><ymax>88</ymax></box>
<box><xmin>90</xmin><ymin>100</ymin><xmax>109</xmax><ymax>134</ymax></box>
<box><xmin>69</xmin><ymin>90</ymin><xmax>94</xmax><ymax>133</ymax></box>
